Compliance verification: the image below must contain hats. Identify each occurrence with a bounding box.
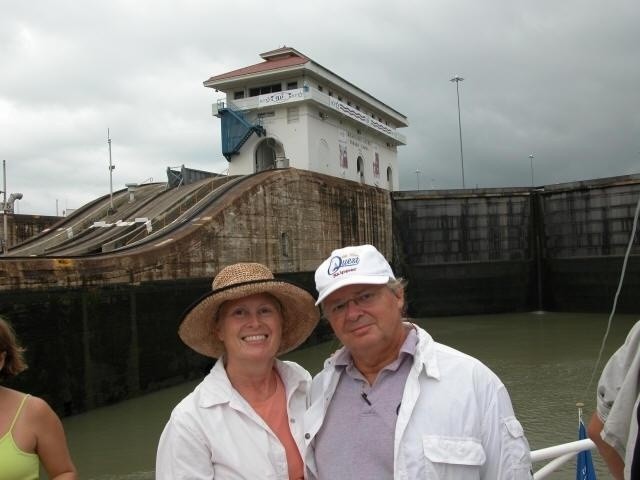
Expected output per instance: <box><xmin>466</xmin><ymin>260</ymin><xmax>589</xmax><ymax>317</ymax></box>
<box><xmin>176</xmin><ymin>261</ymin><xmax>322</xmax><ymax>361</ymax></box>
<box><xmin>313</xmin><ymin>243</ymin><xmax>397</xmax><ymax>310</ymax></box>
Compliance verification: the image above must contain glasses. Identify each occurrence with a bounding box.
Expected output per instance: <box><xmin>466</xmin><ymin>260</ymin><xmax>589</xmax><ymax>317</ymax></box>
<box><xmin>329</xmin><ymin>291</ymin><xmax>379</xmax><ymax>318</ymax></box>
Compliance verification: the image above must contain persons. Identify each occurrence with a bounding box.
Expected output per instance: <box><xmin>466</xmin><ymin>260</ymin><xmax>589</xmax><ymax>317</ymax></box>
<box><xmin>154</xmin><ymin>261</ymin><xmax>346</xmax><ymax>480</ymax></box>
<box><xmin>0</xmin><ymin>317</ymin><xmax>80</xmax><ymax>480</ymax></box>
<box><xmin>586</xmin><ymin>318</ymin><xmax>640</xmax><ymax>480</ymax></box>
<box><xmin>303</xmin><ymin>244</ymin><xmax>534</xmax><ymax>480</ymax></box>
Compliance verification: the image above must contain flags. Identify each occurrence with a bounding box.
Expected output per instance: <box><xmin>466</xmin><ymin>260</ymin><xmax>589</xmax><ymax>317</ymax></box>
<box><xmin>576</xmin><ymin>418</ymin><xmax>599</xmax><ymax>480</ymax></box>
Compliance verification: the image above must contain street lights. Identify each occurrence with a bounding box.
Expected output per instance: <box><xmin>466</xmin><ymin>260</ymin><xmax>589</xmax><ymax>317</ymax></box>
<box><xmin>529</xmin><ymin>152</ymin><xmax>536</xmax><ymax>187</ymax></box>
<box><xmin>449</xmin><ymin>75</ymin><xmax>465</xmax><ymax>190</ymax></box>
<box><xmin>414</xmin><ymin>168</ymin><xmax>422</xmax><ymax>190</ymax></box>
